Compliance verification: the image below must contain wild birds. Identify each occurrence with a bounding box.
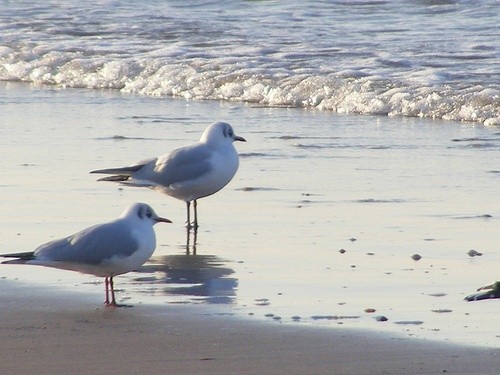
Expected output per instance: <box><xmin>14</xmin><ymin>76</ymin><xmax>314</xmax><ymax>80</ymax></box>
<box><xmin>0</xmin><ymin>202</ymin><xmax>175</xmax><ymax>308</ymax></box>
<box><xmin>89</xmin><ymin>122</ymin><xmax>247</xmax><ymax>228</ymax></box>
